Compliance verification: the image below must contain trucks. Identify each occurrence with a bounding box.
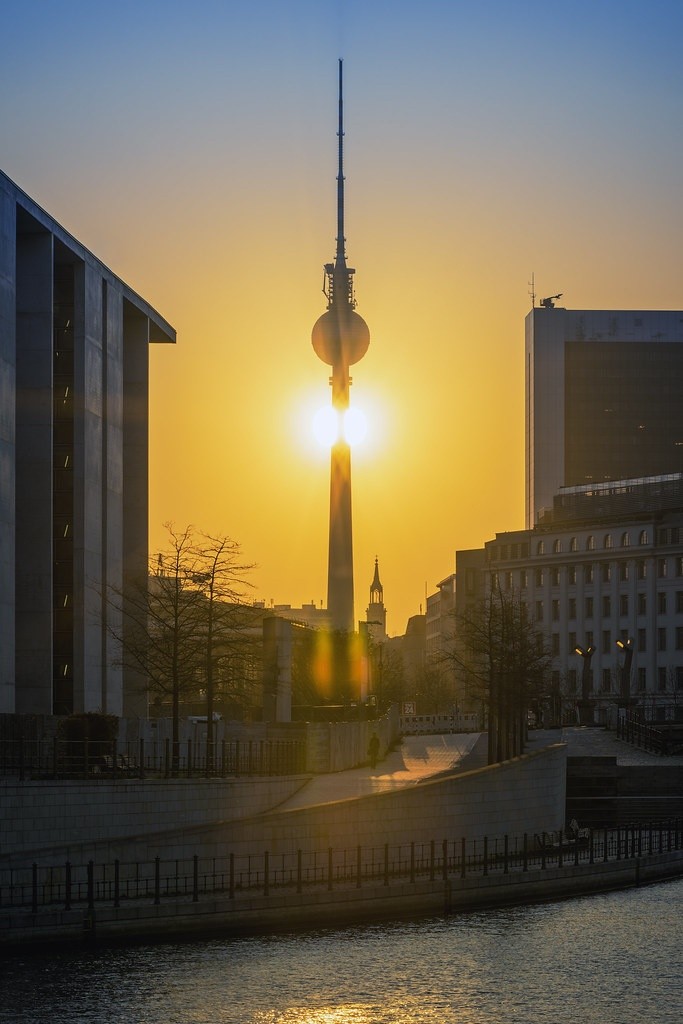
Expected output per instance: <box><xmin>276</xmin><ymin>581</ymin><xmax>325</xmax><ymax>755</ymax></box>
<box><xmin>187</xmin><ymin>711</ymin><xmax>224</xmax><ymax>724</ymax></box>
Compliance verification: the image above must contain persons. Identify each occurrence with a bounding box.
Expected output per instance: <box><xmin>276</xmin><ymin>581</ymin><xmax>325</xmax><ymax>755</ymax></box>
<box><xmin>369</xmin><ymin>732</ymin><xmax>380</xmax><ymax>769</ymax></box>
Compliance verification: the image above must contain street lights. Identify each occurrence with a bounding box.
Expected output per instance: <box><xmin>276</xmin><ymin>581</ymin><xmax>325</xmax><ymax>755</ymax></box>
<box><xmin>574</xmin><ymin>644</ymin><xmax>596</xmax><ymax>700</ymax></box>
<box><xmin>615</xmin><ymin>637</ymin><xmax>634</xmax><ymax>705</ymax></box>
